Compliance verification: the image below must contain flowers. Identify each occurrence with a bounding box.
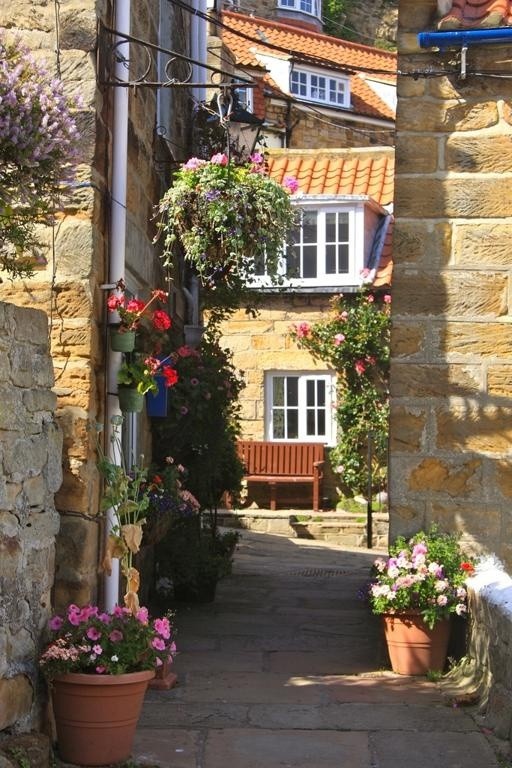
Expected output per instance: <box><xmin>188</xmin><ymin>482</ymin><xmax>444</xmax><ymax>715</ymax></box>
<box><xmin>148</xmin><ymin>149</ymin><xmax>298</xmax><ymax>292</ymax></box>
<box><xmin>130</xmin><ymin>455</ymin><xmax>200</xmax><ymax>544</ymax></box>
<box><xmin>0</xmin><ymin>39</ymin><xmax>85</xmax><ymax>278</ymax></box>
<box><xmin>37</xmin><ymin>601</ymin><xmax>176</xmax><ymax>677</ymax></box>
<box><xmin>118</xmin><ymin>346</ymin><xmax>195</xmax><ymax>394</ymax></box>
<box><xmin>108</xmin><ymin>290</ymin><xmax>173</xmax><ymax>331</ymax></box>
<box><xmin>367</xmin><ymin>529</ymin><xmax>470</xmax><ymax>627</ymax></box>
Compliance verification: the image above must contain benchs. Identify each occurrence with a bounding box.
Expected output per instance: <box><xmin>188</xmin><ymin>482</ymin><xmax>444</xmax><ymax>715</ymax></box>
<box><xmin>226</xmin><ymin>442</ymin><xmax>328</xmax><ymax>512</ymax></box>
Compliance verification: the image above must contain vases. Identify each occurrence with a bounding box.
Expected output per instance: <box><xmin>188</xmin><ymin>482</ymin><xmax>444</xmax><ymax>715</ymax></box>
<box><xmin>109</xmin><ymin>325</ymin><xmax>135</xmax><ymax>349</ymax></box>
<box><xmin>119</xmin><ymin>385</ymin><xmax>143</xmax><ymax>412</ymax></box>
<box><xmin>380</xmin><ymin>607</ymin><xmax>451</xmax><ymax>677</ymax></box>
<box><xmin>45</xmin><ymin>672</ymin><xmax>150</xmax><ymax>765</ymax></box>
